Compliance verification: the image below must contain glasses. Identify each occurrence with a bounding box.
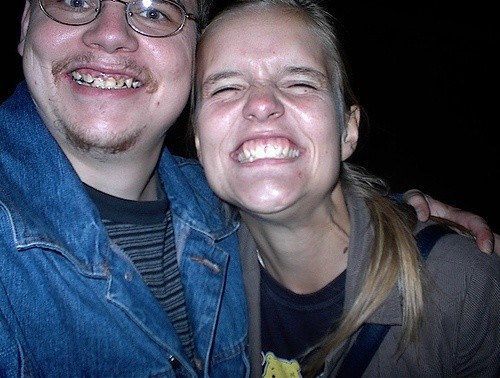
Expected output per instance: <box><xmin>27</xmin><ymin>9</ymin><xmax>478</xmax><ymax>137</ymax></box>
<box><xmin>39</xmin><ymin>0</ymin><xmax>200</xmax><ymax>41</ymax></box>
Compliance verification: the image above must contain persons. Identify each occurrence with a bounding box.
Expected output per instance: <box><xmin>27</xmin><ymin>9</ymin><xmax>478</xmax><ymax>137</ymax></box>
<box><xmin>191</xmin><ymin>0</ymin><xmax>499</xmax><ymax>378</ymax></box>
<box><xmin>0</xmin><ymin>0</ymin><xmax>500</xmax><ymax>378</ymax></box>
